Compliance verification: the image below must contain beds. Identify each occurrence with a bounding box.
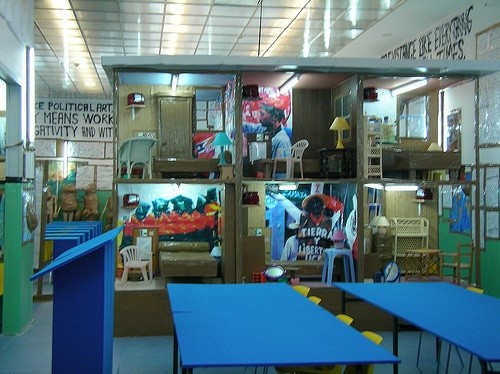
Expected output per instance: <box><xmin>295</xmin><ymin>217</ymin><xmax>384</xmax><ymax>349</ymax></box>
<box><xmin>158</xmin><ymin>241</ymin><xmax>217</xmax><ymax>277</ymax></box>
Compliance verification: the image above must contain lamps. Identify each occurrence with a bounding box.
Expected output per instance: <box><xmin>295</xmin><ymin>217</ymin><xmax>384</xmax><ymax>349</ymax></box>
<box><xmin>278</xmin><ymin>73</ymin><xmax>302</xmax><ymax>95</ymax></box>
<box><xmin>209</xmin><ymin>132</ymin><xmax>233</xmax><ymax>165</ymax></box>
<box><xmin>426</xmin><ymin>142</ymin><xmax>442</xmax><ymax>151</ymax></box>
<box><xmin>384</xmin><ymin>182</ymin><xmax>419</xmax><ymax>192</ymax></box>
<box><xmin>277</xmin><ymin>182</ymin><xmax>299</xmax><ymax>191</ymax></box>
<box><xmin>170</xmin><ymin>74</ymin><xmax>178</xmax><ymax>90</ymax></box>
<box><xmin>369</xmin><ymin>215</ymin><xmax>390</xmax><ymax>234</ymax></box>
<box><xmin>329</xmin><ymin>116</ymin><xmax>351</xmax><ymax>148</ymax></box>
<box><xmin>211</xmin><ymin>245</ymin><xmax>222</xmax><ymax>257</ymax></box>
<box><xmin>24</xmin><ymin>45</ymin><xmax>35</xmax><ymax>152</ymax></box>
<box><xmin>390</xmin><ymin>78</ymin><xmax>429</xmax><ymax>98</ymax></box>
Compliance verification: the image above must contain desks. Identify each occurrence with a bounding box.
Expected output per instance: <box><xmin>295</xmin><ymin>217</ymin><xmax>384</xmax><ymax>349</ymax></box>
<box><xmin>216</xmin><ymin>163</ymin><xmax>235</xmax><ymax>179</ymax></box>
<box><xmin>404</xmin><ymin>249</ymin><xmax>440</xmax><ymax>282</ymax></box>
<box><xmin>165</xmin><ymin>282</ymin><xmax>401</xmax><ymax>374</ymax></box>
<box><xmin>327</xmin><ymin>281</ymin><xmax>500</xmax><ymax>374</ymax></box>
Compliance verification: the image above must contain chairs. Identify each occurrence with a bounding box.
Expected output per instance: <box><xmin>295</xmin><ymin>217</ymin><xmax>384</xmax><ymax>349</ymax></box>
<box><xmin>272</xmin><ymin>139</ymin><xmax>309</xmax><ymax>180</ymax></box>
<box><xmin>117</xmin><ymin>138</ymin><xmax>158</xmax><ymax>180</ymax></box>
<box><xmin>275</xmin><ymin>285</ymin><xmax>382</xmax><ymax>374</ymax></box>
<box><xmin>438</xmin><ymin>241</ymin><xmax>474</xmax><ymax>282</ymax></box>
<box><xmin>119</xmin><ymin>244</ymin><xmax>153</xmax><ymax>283</ymax></box>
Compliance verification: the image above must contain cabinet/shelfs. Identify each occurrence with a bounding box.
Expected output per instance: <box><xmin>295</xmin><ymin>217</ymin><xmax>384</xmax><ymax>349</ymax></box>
<box><xmin>362</xmin><ymin>115</ymin><xmax>383</xmax><ymax>179</ymax></box>
<box><xmin>317</xmin><ymin>147</ymin><xmax>356</xmax><ymax>179</ymax></box>
<box><xmin>244</xmin><ymin>203</ymin><xmax>271</xmax><ymax>275</ymax></box>
<box><xmin>390</xmin><ymin>216</ymin><xmax>429</xmax><ymax>264</ymax></box>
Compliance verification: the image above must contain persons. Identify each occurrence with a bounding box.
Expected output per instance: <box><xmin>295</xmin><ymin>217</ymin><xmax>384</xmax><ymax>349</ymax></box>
<box><xmin>258</xmin><ymin>94</ymin><xmax>294</xmax><ymax>180</ymax></box>
<box><xmin>279</xmin><ymin>193</ymin><xmax>345</xmax><ymax>261</ymax></box>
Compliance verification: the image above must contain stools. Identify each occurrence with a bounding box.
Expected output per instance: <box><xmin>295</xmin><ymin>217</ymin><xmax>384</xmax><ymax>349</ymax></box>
<box><xmin>321</xmin><ymin>247</ymin><xmax>356</xmax><ymax>287</ymax></box>
